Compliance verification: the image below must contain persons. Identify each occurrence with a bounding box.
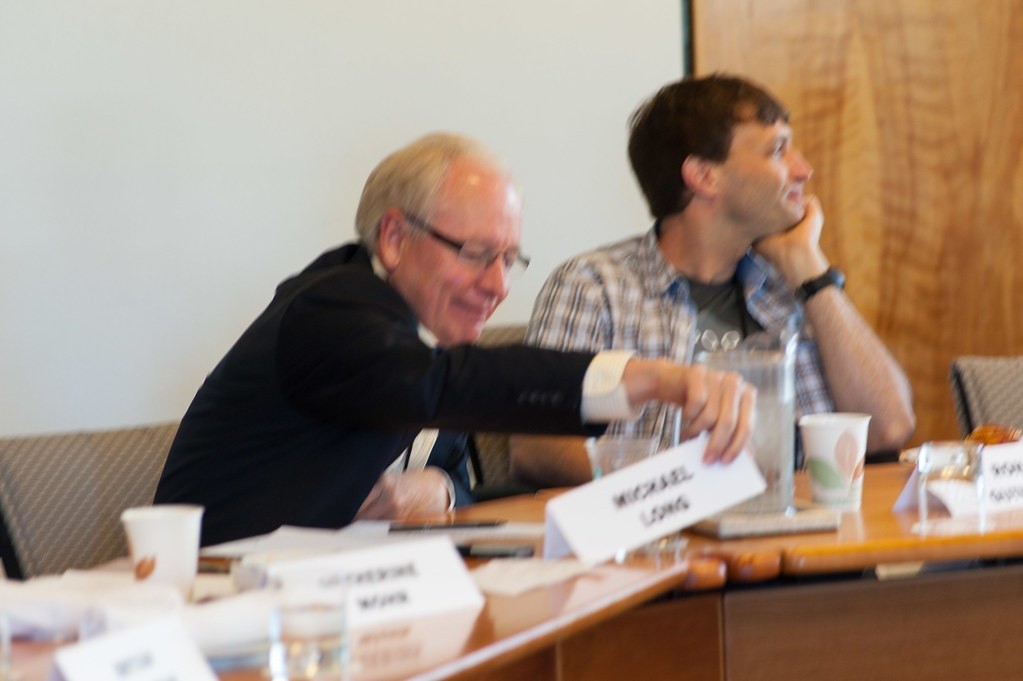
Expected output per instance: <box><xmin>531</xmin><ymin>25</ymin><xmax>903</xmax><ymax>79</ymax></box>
<box><xmin>514</xmin><ymin>74</ymin><xmax>916</xmax><ymax>488</ymax></box>
<box><xmin>153</xmin><ymin>130</ymin><xmax>754</xmax><ymax>525</ymax></box>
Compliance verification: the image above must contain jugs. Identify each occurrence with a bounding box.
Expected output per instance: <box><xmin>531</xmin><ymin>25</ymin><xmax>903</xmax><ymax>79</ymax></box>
<box><xmin>695</xmin><ymin>332</ymin><xmax>798</xmax><ymax>514</ymax></box>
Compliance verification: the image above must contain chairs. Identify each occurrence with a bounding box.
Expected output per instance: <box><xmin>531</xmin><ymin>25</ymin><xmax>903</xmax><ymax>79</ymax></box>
<box><xmin>949</xmin><ymin>354</ymin><xmax>1023</xmax><ymax>440</ymax></box>
<box><xmin>0</xmin><ymin>422</ymin><xmax>181</xmax><ymax>580</ymax></box>
<box><xmin>468</xmin><ymin>327</ymin><xmax>530</xmax><ymax>487</ymax></box>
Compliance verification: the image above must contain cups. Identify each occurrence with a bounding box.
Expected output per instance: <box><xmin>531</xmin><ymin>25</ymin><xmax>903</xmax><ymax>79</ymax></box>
<box><xmin>269</xmin><ymin>602</ymin><xmax>345</xmax><ymax>681</ymax></box>
<box><xmin>120</xmin><ymin>504</ymin><xmax>204</xmax><ymax>602</ymax></box>
<box><xmin>585</xmin><ymin>436</ymin><xmax>661</xmax><ymax>480</ymax></box>
<box><xmin>916</xmin><ymin>441</ymin><xmax>988</xmax><ymax>536</ymax></box>
<box><xmin>799</xmin><ymin>411</ymin><xmax>872</xmax><ymax>511</ymax></box>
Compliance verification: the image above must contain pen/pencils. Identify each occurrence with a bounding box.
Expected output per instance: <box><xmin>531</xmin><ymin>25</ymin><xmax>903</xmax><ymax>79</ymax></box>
<box><xmin>456</xmin><ymin>545</ymin><xmax>532</xmax><ymax>559</ymax></box>
<box><xmin>389</xmin><ymin>517</ymin><xmax>507</xmax><ymax>533</ymax></box>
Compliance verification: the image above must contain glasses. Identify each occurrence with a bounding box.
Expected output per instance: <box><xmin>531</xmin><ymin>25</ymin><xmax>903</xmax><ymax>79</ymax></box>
<box><xmin>402</xmin><ymin>211</ymin><xmax>530</xmax><ymax>274</ymax></box>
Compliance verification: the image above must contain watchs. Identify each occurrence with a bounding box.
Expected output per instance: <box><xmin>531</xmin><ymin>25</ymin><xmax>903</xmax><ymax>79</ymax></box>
<box><xmin>796</xmin><ymin>266</ymin><xmax>845</xmax><ymax>300</ymax></box>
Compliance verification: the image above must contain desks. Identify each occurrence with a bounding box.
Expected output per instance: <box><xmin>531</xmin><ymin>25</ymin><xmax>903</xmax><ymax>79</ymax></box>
<box><xmin>0</xmin><ymin>465</ymin><xmax>1023</xmax><ymax>681</ymax></box>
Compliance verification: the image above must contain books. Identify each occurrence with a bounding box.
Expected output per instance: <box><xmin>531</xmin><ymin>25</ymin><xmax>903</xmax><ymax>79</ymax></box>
<box><xmin>197</xmin><ymin>525</ymin><xmax>296</xmax><ymax>575</ymax></box>
<box><xmin>692</xmin><ymin>498</ymin><xmax>842</xmax><ymax>540</ymax></box>
